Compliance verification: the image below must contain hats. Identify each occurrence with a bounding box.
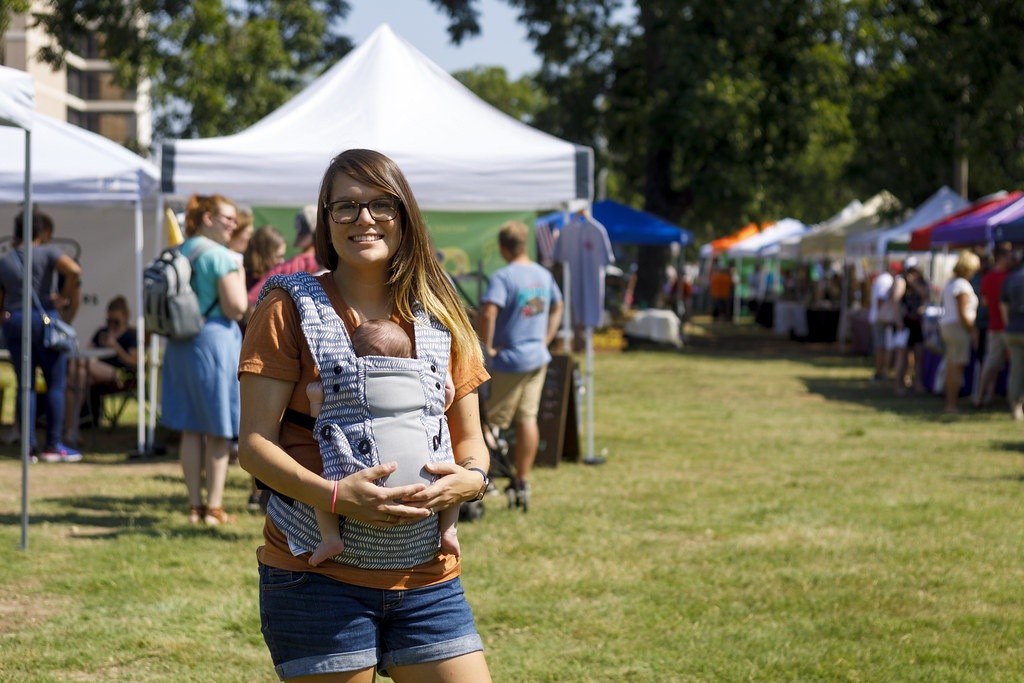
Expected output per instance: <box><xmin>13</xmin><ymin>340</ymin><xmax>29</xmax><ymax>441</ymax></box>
<box><xmin>294</xmin><ymin>205</ymin><xmax>318</xmax><ymax>247</ymax></box>
<box><xmin>889</xmin><ymin>261</ymin><xmax>905</xmax><ymax>274</ymax></box>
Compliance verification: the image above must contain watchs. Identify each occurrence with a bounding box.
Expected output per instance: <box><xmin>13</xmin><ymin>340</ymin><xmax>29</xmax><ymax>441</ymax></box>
<box><xmin>466</xmin><ymin>467</ymin><xmax>489</xmax><ymax>502</ymax></box>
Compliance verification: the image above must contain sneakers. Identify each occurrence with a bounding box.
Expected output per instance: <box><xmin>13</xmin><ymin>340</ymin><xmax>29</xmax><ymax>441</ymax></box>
<box><xmin>19</xmin><ymin>449</ymin><xmax>37</xmax><ymax>462</ymax></box>
<box><xmin>40</xmin><ymin>442</ymin><xmax>82</xmax><ymax>463</ymax></box>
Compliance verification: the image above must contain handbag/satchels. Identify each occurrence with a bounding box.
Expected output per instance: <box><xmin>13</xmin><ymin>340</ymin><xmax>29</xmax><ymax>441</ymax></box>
<box><xmin>44</xmin><ymin>317</ymin><xmax>78</xmax><ymax>356</ymax></box>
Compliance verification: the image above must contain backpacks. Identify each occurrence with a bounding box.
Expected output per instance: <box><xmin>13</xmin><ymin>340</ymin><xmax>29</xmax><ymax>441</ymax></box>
<box><xmin>143</xmin><ymin>238</ymin><xmax>219</xmax><ymax>343</ymax></box>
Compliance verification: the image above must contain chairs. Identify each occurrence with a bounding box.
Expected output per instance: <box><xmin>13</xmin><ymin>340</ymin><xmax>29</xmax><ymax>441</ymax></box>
<box><xmin>88</xmin><ymin>356</ymin><xmax>157</xmax><ymax>431</ymax></box>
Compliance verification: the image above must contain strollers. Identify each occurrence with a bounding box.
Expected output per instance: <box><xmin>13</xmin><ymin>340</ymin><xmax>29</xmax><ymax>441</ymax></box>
<box><xmin>458</xmin><ymin>379</ymin><xmax>532</xmax><ymax>521</ymax></box>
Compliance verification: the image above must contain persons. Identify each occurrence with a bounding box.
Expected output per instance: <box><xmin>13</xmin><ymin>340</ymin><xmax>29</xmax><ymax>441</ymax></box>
<box><xmin>237</xmin><ymin>148</ymin><xmax>493</xmax><ymax>683</ymax></box>
<box><xmin>156</xmin><ymin>193</ymin><xmax>248</xmax><ymax>528</ymax></box>
<box><xmin>0</xmin><ymin>204</ymin><xmax>331</xmax><ymax>513</ymax></box>
<box><xmin>307</xmin><ymin>319</ymin><xmax>460</xmax><ymax>567</ymax></box>
<box><xmin>708</xmin><ymin>243</ymin><xmax>1022</xmax><ymax>423</ymax></box>
<box><xmin>481</xmin><ymin>218</ymin><xmax>562</xmax><ymax>502</ymax></box>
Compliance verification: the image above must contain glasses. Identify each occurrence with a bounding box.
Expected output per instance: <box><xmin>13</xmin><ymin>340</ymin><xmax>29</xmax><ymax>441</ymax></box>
<box><xmin>106</xmin><ymin>319</ymin><xmax>122</xmax><ymax>325</ymax></box>
<box><xmin>216</xmin><ymin>212</ymin><xmax>238</xmax><ymax>225</ymax></box>
<box><xmin>323</xmin><ymin>198</ymin><xmax>401</xmax><ymax>224</ymax></box>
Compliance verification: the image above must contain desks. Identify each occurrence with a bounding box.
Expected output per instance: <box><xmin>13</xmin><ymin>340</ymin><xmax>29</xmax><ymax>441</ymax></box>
<box><xmin>0</xmin><ymin>349</ymin><xmax>115</xmax><ymax>444</ymax></box>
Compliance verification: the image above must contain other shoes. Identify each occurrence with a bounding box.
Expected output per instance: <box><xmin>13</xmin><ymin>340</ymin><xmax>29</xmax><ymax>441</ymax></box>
<box><xmin>873</xmin><ymin>375</ymin><xmax>996</xmax><ymax>414</ymax></box>
<box><xmin>504</xmin><ymin>480</ymin><xmax>530</xmax><ymax>496</ymax></box>
<box><xmin>485</xmin><ymin>482</ymin><xmax>499</xmax><ymax>497</ymax></box>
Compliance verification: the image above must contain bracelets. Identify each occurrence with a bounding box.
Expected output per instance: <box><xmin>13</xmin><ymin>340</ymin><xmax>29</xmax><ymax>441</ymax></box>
<box><xmin>330</xmin><ymin>478</ymin><xmax>338</xmax><ymax>514</ymax></box>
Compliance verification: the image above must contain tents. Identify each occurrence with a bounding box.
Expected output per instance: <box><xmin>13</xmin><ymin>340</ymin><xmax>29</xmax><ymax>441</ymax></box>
<box><xmin>148</xmin><ymin>20</ymin><xmax>595</xmax><ymax>459</ymax></box>
<box><xmin>592</xmin><ymin>198</ymin><xmax>689</xmax><ymax>319</ymax></box>
<box><xmin>697</xmin><ymin>185</ymin><xmax>1024</xmax><ymax>346</ymax></box>
<box><xmin>0</xmin><ymin>113</ymin><xmax>160</xmax><ymax>458</ymax></box>
<box><xmin>0</xmin><ymin>62</ymin><xmax>36</xmax><ymax>550</ymax></box>
